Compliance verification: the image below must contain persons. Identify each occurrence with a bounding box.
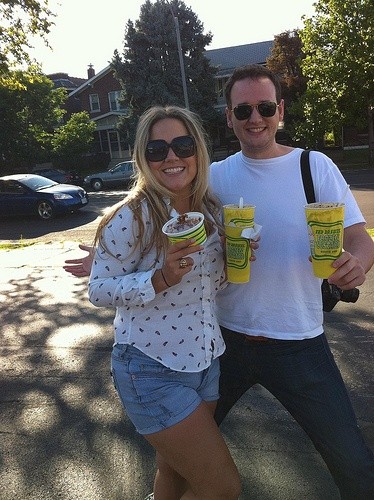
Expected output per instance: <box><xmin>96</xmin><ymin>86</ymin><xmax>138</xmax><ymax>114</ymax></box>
<box><xmin>62</xmin><ymin>66</ymin><xmax>374</xmax><ymax>500</ymax></box>
<box><xmin>88</xmin><ymin>104</ymin><xmax>261</xmax><ymax>499</ymax></box>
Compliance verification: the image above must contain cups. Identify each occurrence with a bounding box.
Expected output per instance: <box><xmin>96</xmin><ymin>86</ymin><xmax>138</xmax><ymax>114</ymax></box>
<box><xmin>223</xmin><ymin>204</ymin><xmax>256</xmax><ymax>284</ymax></box>
<box><xmin>304</xmin><ymin>202</ymin><xmax>344</xmax><ymax>279</ymax></box>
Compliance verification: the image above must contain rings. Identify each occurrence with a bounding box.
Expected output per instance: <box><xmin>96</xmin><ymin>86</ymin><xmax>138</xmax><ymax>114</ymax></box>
<box><xmin>180</xmin><ymin>258</ymin><xmax>188</xmax><ymax>268</ymax></box>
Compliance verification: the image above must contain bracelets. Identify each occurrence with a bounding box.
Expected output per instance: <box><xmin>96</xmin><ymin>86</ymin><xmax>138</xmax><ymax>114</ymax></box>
<box><xmin>161</xmin><ymin>269</ymin><xmax>171</xmax><ymax>289</ymax></box>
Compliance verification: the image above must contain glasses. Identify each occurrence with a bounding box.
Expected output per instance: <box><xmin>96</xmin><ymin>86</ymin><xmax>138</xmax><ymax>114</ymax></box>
<box><xmin>145</xmin><ymin>135</ymin><xmax>196</xmax><ymax>162</ymax></box>
<box><xmin>231</xmin><ymin>102</ymin><xmax>280</xmax><ymax>120</ymax></box>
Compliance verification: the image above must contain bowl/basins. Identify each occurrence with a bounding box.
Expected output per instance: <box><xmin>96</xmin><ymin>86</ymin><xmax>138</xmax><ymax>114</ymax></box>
<box><xmin>161</xmin><ymin>211</ymin><xmax>208</xmax><ymax>247</ymax></box>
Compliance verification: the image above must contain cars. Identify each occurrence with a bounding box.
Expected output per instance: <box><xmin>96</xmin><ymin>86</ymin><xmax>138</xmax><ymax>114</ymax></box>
<box><xmin>83</xmin><ymin>160</ymin><xmax>137</xmax><ymax>191</ymax></box>
<box><xmin>0</xmin><ymin>167</ymin><xmax>89</xmax><ymax>221</ymax></box>
